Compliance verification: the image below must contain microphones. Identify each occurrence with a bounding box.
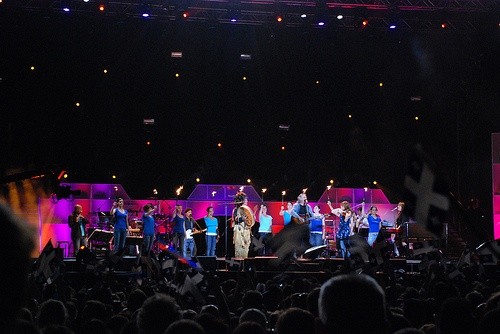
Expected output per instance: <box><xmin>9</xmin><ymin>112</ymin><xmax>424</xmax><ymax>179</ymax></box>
<box><xmin>392</xmin><ymin>207</ymin><xmax>398</xmax><ymax>211</ymax></box>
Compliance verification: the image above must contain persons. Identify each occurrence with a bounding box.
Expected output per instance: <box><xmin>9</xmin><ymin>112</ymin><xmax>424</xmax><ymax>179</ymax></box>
<box><xmin>0</xmin><ymin>192</ymin><xmax>500</xmax><ymax>334</ymax></box>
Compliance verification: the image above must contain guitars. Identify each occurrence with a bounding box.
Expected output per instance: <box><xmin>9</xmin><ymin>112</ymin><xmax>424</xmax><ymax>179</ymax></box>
<box><xmin>291</xmin><ymin>213</ymin><xmax>331</xmax><ymax>225</ymax></box>
<box><xmin>186</xmin><ymin>228</ymin><xmax>207</xmax><ymax>239</ymax></box>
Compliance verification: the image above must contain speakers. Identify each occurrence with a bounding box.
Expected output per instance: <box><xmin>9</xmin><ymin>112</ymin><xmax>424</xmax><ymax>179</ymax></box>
<box><xmin>60</xmin><ymin>258</ymin><xmax>76</xmax><ymax>272</ymax></box>
<box><xmin>386</xmin><ymin>258</ymin><xmax>406</xmax><ymax>272</ymax></box>
<box><xmin>113</xmin><ymin>255</ymin><xmax>138</xmax><ymax>270</ymax></box>
<box><xmin>192</xmin><ymin>256</ymin><xmax>217</xmax><ymax>270</ymax></box>
<box><xmin>243</xmin><ymin>258</ymin><xmax>258</xmax><ymax>272</ymax></box>
